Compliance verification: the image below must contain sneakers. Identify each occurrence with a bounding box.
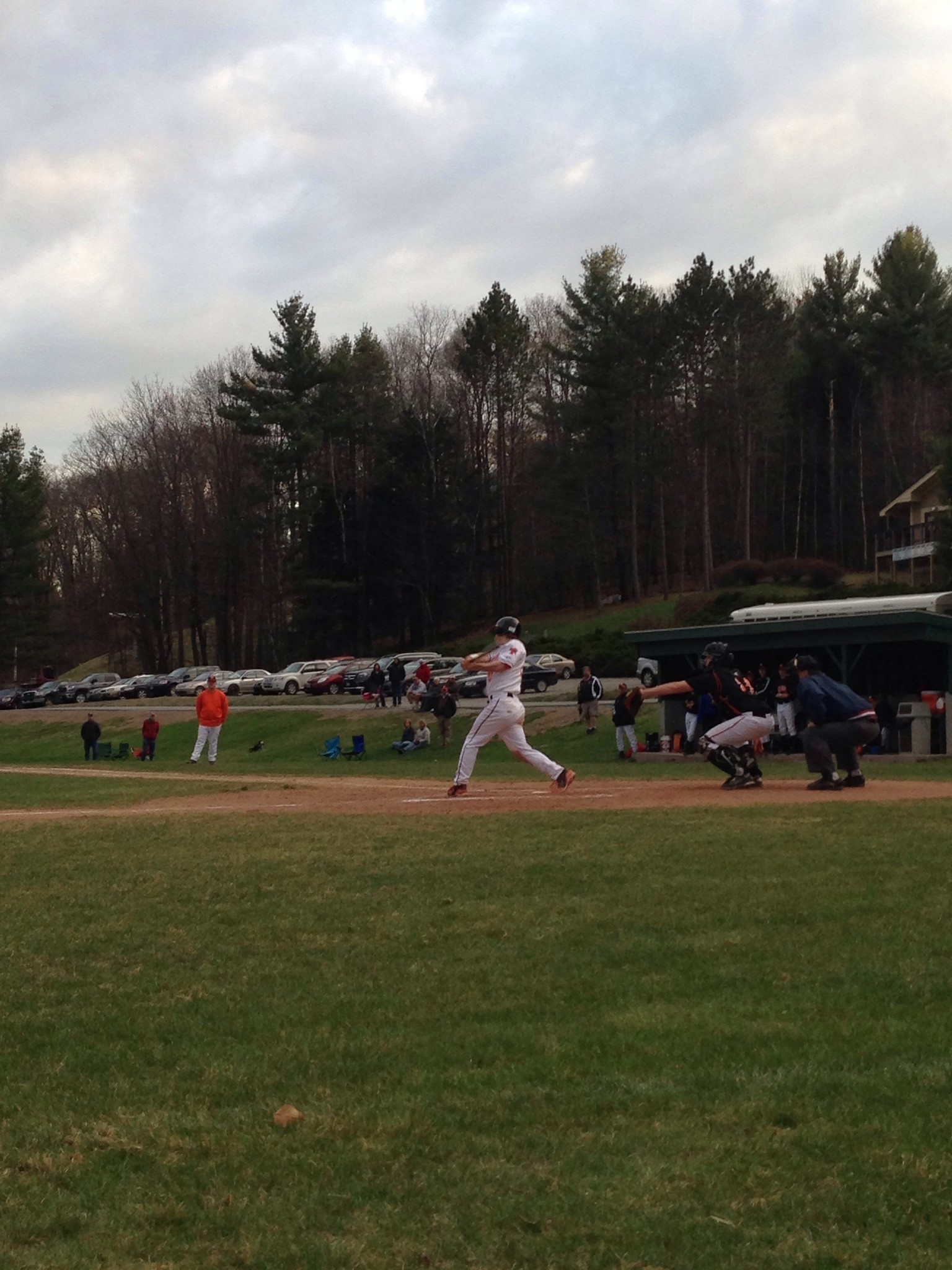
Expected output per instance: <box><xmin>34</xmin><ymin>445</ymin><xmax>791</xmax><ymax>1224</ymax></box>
<box><xmin>447</xmin><ymin>783</ymin><xmax>468</xmax><ymax>796</ymax></box>
<box><xmin>842</xmin><ymin>774</ymin><xmax>865</xmax><ymax>787</ymax></box>
<box><xmin>752</xmin><ymin>769</ymin><xmax>763</xmax><ymax>784</ymax></box>
<box><xmin>807</xmin><ymin>776</ymin><xmax>843</xmax><ymax>790</ymax></box>
<box><xmin>721</xmin><ymin>772</ymin><xmax>755</xmax><ymax>789</ymax></box>
<box><xmin>556</xmin><ymin>769</ymin><xmax>576</xmax><ymax>792</ymax></box>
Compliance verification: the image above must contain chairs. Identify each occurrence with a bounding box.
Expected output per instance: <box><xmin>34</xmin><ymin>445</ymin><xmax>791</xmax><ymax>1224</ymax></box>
<box><xmin>321</xmin><ymin>734</ymin><xmax>346</xmax><ymax>761</ymax></box>
<box><xmin>342</xmin><ymin>734</ymin><xmax>366</xmax><ymax>760</ymax></box>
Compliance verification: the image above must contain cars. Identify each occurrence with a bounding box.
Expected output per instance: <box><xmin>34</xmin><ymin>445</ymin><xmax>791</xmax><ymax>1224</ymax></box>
<box><xmin>1</xmin><ymin>649</ymin><xmax>575</xmax><ymax>711</ymax></box>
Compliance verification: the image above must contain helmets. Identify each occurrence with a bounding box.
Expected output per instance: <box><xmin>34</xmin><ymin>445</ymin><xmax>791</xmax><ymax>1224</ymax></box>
<box><xmin>489</xmin><ymin>616</ymin><xmax>522</xmax><ymax>639</ymax></box>
<box><xmin>702</xmin><ymin>642</ymin><xmax>734</xmax><ymax>664</ymax></box>
<box><xmin>793</xmin><ymin>655</ymin><xmax>818</xmax><ymax>670</ymax></box>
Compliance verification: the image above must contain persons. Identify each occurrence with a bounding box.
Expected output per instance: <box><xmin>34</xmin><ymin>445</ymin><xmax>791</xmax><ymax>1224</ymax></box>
<box><xmin>577</xmin><ymin>665</ymin><xmax>603</xmax><ymax>734</ymax></box>
<box><xmin>434</xmin><ymin>684</ymin><xmax>456</xmax><ymax>746</ymax></box>
<box><xmin>392</xmin><ymin>721</ymin><xmax>415</xmax><ymax>751</ymax></box>
<box><xmin>446</xmin><ymin>617</ymin><xmax>576</xmax><ymax>798</ymax></box>
<box><xmin>81</xmin><ymin>712</ymin><xmax>102</xmax><ymax>761</ymax></box>
<box><xmin>389</xmin><ymin>656</ymin><xmax>406</xmax><ymax>706</ymax></box>
<box><xmin>625</xmin><ymin>642</ymin><xmax>775</xmax><ymax>788</ymax></box>
<box><xmin>398</xmin><ymin>720</ymin><xmax>431</xmax><ymax>754</ymax></box>
<box><xmin>368</xmin><ymin>663</ymin><xmax>386</xmax><ymax>708</ymax></box>
<box><xmin>189</xmin><ymin>676</ymin><xmax>229</xmax><ymax>764</ymax></box>
<box><xmin>684</xmin><ymin>660</ymin><xmax>892</xmax><ymax>752</ymax></box>
<box><xmin>140</xmin><ymin>714</ymin><xmax>159</xmax><ymax>761</ymax></box>
<box><xmin>613</xmin><ymin>683</ymin><xmax>639</xmax><ymax>759</ymax></box>
<box><xmin>790</xmin><ymin>655</ymin><xmax>880</xmax><ymax>789</ymax></box>
<box><xmin>405</xmin><ymin>658</ymin><xmax>458</xmax><ymax>712</ymax></box>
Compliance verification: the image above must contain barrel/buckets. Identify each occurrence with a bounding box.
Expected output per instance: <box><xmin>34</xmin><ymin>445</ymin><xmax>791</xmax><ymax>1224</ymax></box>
<box><xmin>660</xmin><ymin>735</ymin><xmax>671</xmax><ymax>753</ymax></box>
<box><xmin>920</xmin><ymin>690</ymin><xmax>941</xmax><ymax>712</ymax></box>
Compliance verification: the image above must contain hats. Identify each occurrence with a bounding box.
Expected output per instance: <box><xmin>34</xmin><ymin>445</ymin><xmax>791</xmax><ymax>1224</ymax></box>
<box><xmin>758</xmin><ymin>662</ymin><xmax>766</xmax><ymax>669</ymax></box>
<box><xmin>88</xmin><ymin>713</ymin><xmax>92</xmax><ymax>717</ymax></box>
<box><xmin>208</xmin><ymin>675</ymin><xmax>217</xmax><ymax>682</ymax></box>
<box><xmin>778</xmin><ymin>664</ymin><xmax>786</xmax><ymax>670</ymax></box>
<box><xmin>150</xmin><ymin>712</ymin><xmax>155</xmax><ymax>717</ymax></box>
<box><xmin>619</xmin><ymin>683</ymin><xmax>627</xmax><ymax>689</ymax></box>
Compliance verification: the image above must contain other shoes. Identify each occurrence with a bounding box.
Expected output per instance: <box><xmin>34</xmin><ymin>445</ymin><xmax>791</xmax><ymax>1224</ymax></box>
<box><xmin>209</xmin><ymin>761</ymin><xmax>215</xmax><ymax>765</ymax></box>
<box><xmin>187</xmin><ymin>760</ymin><xmax>196</xmax><ymax>764</ymax></box>
<box><xmin>398</xmin><ymin>749</ymin><xmax>404</xmax><ymax>755</ymax></box>
<box><xmin>586</xmin><ymin>728</ymin><xmax>597</xmax><ymax>734</ymax></box>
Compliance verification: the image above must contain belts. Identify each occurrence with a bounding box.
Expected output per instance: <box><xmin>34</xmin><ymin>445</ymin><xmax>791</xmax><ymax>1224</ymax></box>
<box><xmin>488</xmin><ymin>692</ymin><xmax>516</xmax><ymax>701</ymax></box>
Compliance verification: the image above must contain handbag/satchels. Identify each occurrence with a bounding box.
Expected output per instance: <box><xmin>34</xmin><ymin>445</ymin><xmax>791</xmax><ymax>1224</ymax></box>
<box><xmin>645</xmin><ymin>732</ymin><xmax>661</xmax><ymax>753</ymax></box>
